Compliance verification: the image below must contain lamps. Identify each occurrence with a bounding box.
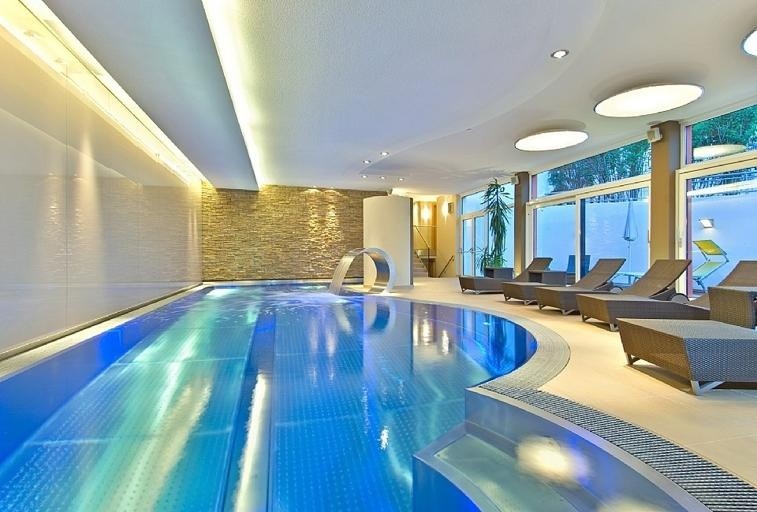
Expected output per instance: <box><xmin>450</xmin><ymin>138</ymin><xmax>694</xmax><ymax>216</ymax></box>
<box><xmin>513</xmin><ymin>82</ymin><xmax>702</xmax><ymax>153</ymax></box>
